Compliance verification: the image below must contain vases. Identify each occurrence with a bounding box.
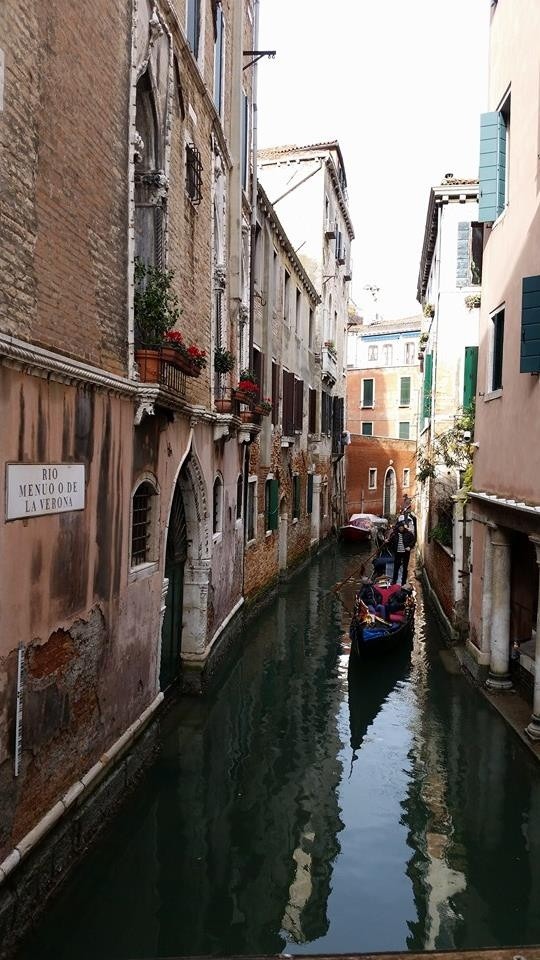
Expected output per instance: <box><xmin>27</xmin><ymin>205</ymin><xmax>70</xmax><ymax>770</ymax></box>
<box><xmin>239</xmin><ymin>411</ymin><xmax>255</xmax><ymax>421</ymax></box>
<box><xmin>161</xmin><ymin>348</ymin><xmax>210</xmax><ymax>379</ymax></box>
<box><xmin>254</xmin><ymin>405</ymin><xmax>270</xmax><ymax>417</ymax></box>
<box><xmin>235</xmin><ymin>390</ymin><xmax>253</xmax><ymax>406</ymax></box>
<box><xmin>214</xmin><ymin>399</ymin><xmax>232</xmax><ymax>413</ymax></box>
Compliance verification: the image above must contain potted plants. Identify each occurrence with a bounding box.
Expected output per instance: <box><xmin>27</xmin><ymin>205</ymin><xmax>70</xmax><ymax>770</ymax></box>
<box><xmin>464</xmin><ymin>292</ymin><xmax>480</xmax><ymax>310</ymax></box>
<box><xmin>423</xmin><ymin>303</ymin><xmax>436</xmax><ymax>318</ymax></box>
<box><xmin>419</xmin><ymin>331</ymin><xmax>430</xmax><ymax>342</ymax></box>
<box><xmin>131</xmin><ymin>253</ymin><xmax>184</xmax><ymax>383</ymax></box>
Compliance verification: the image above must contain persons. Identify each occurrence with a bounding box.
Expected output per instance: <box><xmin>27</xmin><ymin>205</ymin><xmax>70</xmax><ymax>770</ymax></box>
<box><xmin>373</xmin><ymin>528</ymin><xmax>394</xmax><ymax>576</ymax></box>
<box><xmin>359</xmin><ymin>576</ymin><xmax>386</xmax><ymax>619</ymax></box>
<box><xmin>403</xmin><ymin>494</ymin><xmax>412</xmax><ymax>521</ymax></box>
<box><xmin>384</xmin><ymin>584</ymin><xmax>413</xmax><ymax>621</ymax></box>
<box><xmin>384</xmin><ymin>524</ymin><xmax>415</xmax><ymax>586</ymax></box>
<box><xmin>510</xmin><ymin>639</ymin><xmax>521</xmax><ymax>693</ymax></box>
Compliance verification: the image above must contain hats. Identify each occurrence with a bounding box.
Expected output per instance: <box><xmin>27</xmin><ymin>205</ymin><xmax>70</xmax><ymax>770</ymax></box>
<box><xmin>362</xmin><ymin>577</ymin><xmax>372</xmax><ymax>584</ymax></box>
<box><xmin>401</xmin><ymin>583</ymin><xmax>412</xmax><ymax>591</ymax></box>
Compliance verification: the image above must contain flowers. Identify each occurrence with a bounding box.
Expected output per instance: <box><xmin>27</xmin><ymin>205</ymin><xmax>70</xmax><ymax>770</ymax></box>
<box><xmin>256</xmin><ymin>397</ymin><xmax>274</xmax><ymax>410</ymax></box>
<box><xmin>237</xmin><ymin>368</ymin><xmax>259</xmax><ymax>400</ymax></box>
<box><xmin>163</xmin><ymin>329</ymin><xmax>207</xmax><ymax>369</ymax></box>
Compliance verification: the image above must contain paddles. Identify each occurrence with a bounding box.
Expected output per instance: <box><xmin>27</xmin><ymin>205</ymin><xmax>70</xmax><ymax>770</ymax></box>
<box><xmin>332</xmin><ymin>529</ymin><xmax>398</xmax><ymax>593</ymax></box>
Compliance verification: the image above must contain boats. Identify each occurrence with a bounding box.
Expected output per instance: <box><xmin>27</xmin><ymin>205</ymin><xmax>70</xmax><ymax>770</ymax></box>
<box><xmin>393</xmin><ymin>513</ymin><xmax>417</xmax><ymax>534</ymax></box>
<box><xmin>349</xmin><ymin>543</ymin><xmax>416</xmax><ymax>648</ymax></box>
<box><xmin>338</xmin><ymin>514</ymin><xmax>389</xmax><ymax>545</ymax></box>
